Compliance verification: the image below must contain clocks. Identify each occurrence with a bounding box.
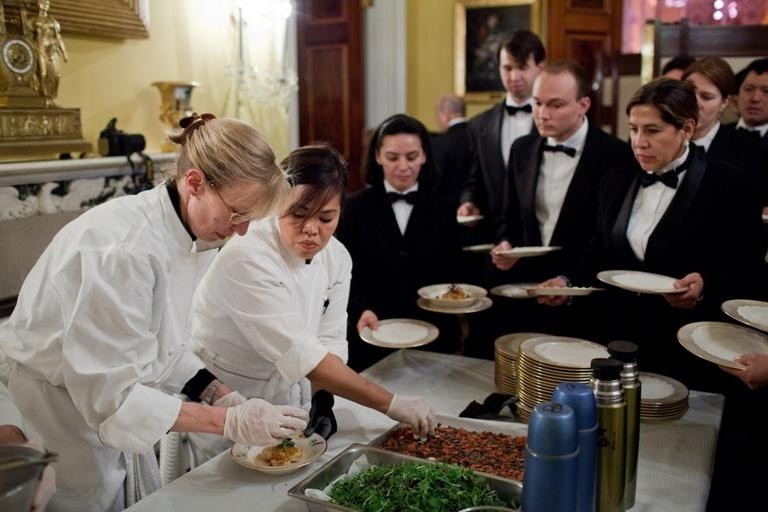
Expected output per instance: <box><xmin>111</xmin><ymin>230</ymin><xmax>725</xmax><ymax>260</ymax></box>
<box><xmin>1</xmin><ymin>35</ymin><xmax>92</xmax><ymax>162</ymax></box>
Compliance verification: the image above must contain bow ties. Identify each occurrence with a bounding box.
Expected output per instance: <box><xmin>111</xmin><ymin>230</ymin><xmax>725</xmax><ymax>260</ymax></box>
<box><xmin>505</xmin><ymin>104</ymin><xmax>532</xmax><ymax>115</ymax></box>
<box><xmin>386</xmin><ymin>190</ymin><xmax>420</xmax><ymax>206</ymax></box>
<box><xmin>642</xmin><ymin>164</ymin><xmax>686</xmax><ymax>190</ymax></box>
<box><xmin>544</xmin><ymin>144</ymin><xmax>576</xmax><ymax>158</ymax></box>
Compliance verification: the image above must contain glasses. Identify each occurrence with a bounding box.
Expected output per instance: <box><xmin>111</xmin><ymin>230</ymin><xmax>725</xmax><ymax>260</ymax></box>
<box><xmin>210</xmin><ymin>182</ymin><xmax>253</xmax><ymax>226</ymax></box>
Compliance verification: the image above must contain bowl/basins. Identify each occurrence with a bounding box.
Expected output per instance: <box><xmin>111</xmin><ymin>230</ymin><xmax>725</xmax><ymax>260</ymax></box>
<box><xmin>419</xmin><ymin>282</ymin><xmax>488</xmax><ymax>307</ymax></box>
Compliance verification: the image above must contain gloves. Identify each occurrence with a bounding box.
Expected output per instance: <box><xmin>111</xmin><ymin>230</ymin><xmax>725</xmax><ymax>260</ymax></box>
<box><xmin>214</xmin><ymin>390</ymin><xmax>310</xmax><ymax>449</ymax></box>
<box><xmin>25</xmin><ymin>438</ymin><xmax>56</xmax><ymax>511</ymax></box>
<box><xmin>304</xmin><ymin>388</ymin><xmax>337</xmax><ymax>442</ymax></box>
<box><xmin>386</xmin><ymin>393</ymin><xmax>437</xmax><ymax>442</ymax></box>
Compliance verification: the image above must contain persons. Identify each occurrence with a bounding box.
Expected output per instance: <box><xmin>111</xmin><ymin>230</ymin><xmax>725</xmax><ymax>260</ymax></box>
<box><xmin>431</xmin><ymin>91</ymin><xmax>473</xmax><ymax>202</ymax></box>
<box><xmin>678</xmin><ymin>58</ymin><xmax>767</xmax><ymax>184</ymax></box>
<box><xmin>1</xmin><ymin>113</ymin><xmax>309</xmax><ymax>511</ymax></box>
<box><xmin>187</xmin><ymin>144</ymin><xmax>439</xmax><ymax>474</ymax></box>
<box><xmin>655</xmin><ymin>54</ymin><xmax>696</xmax><ymax>83</ymax></box>
<box><xmin>733</xmin><ymin>60</ymin><xmax>767</xmax><ymax>140</ymax></box>
<box><xmin>488</xmin><ymin>56</ymin><xmax>618</xmax><ymax>339</ymax></box>
<box><xmin>334</xmin><ymin>115</ymin><xmax>462</xmax><ymax>367</ymax></box>
<box><xmin>719</xmin><ymin>350</ymin><xmax>767</xmax><ymax>389</ymax></box>
<box><xmin>0</xmin><ymin>377</ymin><xmax>57</xmax><ymax>512</ymax></box>
<box><xmin>451</xmin><ymin>31</ymin><xmax>548</xmax><ymax>240</ymax></box>
<box><xmin>30</xmin><ymin>1</ymin><xmax>70</xmax><ymax>99</ymax></box>
<box><xmin>535</xmin><ymin>80</ymin><xmax>767</xmax><ymax>394</ymax></box>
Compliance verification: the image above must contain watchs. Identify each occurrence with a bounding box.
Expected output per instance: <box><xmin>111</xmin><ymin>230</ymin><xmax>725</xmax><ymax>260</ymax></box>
<box><xmin>204</xmin><ymin>374</ymin><xmax>222</xmax><ymax>409</ymax></box>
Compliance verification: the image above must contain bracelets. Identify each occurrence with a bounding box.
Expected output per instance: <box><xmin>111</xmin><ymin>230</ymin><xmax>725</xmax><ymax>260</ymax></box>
<box><xmin>557</xmin><ymin>271</ymin><xmax>575</xmax><ymax>303</ymax></box>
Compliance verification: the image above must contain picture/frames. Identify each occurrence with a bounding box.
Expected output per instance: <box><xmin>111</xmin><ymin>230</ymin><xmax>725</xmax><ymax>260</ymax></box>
<box><xmin>453</xmin><ymin>1</ymin><xmax>541</xmax><ymax>106</ymax></box>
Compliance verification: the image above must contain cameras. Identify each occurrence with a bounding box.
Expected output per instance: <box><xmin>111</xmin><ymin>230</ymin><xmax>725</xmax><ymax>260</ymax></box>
<box><xmin>97</xmin><ymin>118</ymin><xmax>145</xmax><ymax>156</ymax></box>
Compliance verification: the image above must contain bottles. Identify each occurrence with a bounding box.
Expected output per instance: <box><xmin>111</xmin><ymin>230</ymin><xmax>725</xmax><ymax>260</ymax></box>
<box><xmin>522</xmin><ymin>338</ymin><xmax>642</xmax><ymax>511</ymax></box>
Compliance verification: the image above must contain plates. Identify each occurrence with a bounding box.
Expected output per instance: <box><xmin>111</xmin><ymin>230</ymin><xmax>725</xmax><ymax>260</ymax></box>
<box><xmin>229</xmin><ymin>430</ymin><xmax>327</xmax><ymax>476</ymax></box>
<box><xmin>488</xmin><ymin>330</ymin><xmax>689</xmax><ymax>427</ymax></box>
<box><xmin>519</xmin><ymin>283</ymin><xmax>600</xmax><ymax>300</ymax></box>
<box><xmin>495</xmin><ymin>245</ymin><xmax>562</xmax><ymax>258</ymax></box>
<box><xmin>716</xmin><ymin>295</ymin><xmax>767</xmax><ymax>337</ymax></box>
<box><xmin>360</xmin><ymin>316</ymin><xmax>440</xmax><ymax>350</ymax></box>
<box><xmin>595</xmin><ymin>267</ymin><xmax>686</xmax><ymax>297</ymax></box>
<box><xmin>415</xmin><ymin>298</ymin><xmax>494</xmax><ymax>314</ymax></box>
<box><xmin>461</xmin><ymin>243</ymin><xmax>499</xmax><ymax>254</ymax></box>
<box><xmin>677</xmin><ymin>320</ymin><xmax>768</xmax><ymax>371</ymax></box>
<box><xmin>492</xmin><ymin>281</ymin><xmax>527</xmax><ymax>299</ymax></box>
